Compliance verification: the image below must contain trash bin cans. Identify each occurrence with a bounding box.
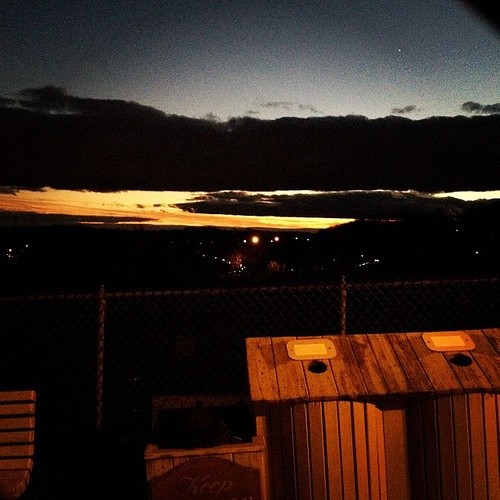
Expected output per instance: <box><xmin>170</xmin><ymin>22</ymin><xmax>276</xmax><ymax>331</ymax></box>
<box><xmin>243</xmin><ymin>326</ymin><xmax>500</xmax><ymax>500</ymax></box>
<box><xmin>141</xmin><ymin>392</ymin><xmax>271</xmax><ymax>500</ymax></box>
